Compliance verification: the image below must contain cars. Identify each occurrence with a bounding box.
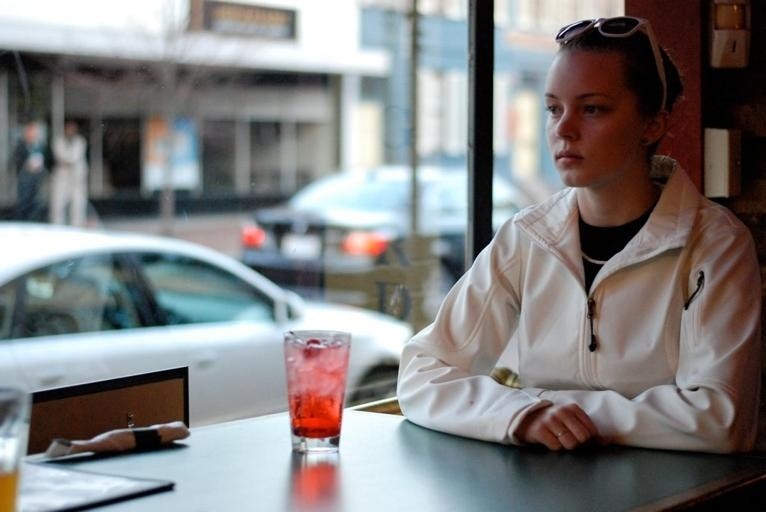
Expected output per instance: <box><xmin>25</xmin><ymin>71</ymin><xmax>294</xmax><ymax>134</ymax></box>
<box><xmin>240</xmin><ymin>163</ymin><xmax>537</xmax><ymax>321</ymax></box>
<box><xmin>0</xmin><ymin>218</ymin><xmax>422</xmax><ymax>455</ymax></box>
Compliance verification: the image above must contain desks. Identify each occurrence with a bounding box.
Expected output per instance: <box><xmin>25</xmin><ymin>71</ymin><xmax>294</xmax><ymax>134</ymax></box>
<box><xmin>22</xmin><ymin>409</ymin><xmax>766</xmax><ymax>512</ymax></box>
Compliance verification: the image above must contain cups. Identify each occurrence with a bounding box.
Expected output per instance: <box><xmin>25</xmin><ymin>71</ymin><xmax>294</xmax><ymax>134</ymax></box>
<box><xmin>0</xmin><ymin>387</ymin><xmax>36</xmax><ymax>510</ymax></box>
<box><xmin>281</xmin><ymin>329</ymin><xmax>351</xmax><ymax>451</ymax></box>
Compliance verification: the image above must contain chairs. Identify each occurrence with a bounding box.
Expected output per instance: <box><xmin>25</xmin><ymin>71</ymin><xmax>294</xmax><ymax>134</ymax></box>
<box><xmin>52</xmin><ymin>278</ymin><xmax>135</xmax><ymax>330</ymax></box>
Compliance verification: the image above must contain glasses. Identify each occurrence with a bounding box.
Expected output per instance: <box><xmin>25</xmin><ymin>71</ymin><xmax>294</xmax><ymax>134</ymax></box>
<box><xmin>556</xmin><ymin>15</ymin><xmax>667</xmax><ymax>111</ymax></box>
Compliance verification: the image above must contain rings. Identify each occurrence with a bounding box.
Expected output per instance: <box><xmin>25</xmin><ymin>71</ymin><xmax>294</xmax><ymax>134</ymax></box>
<box><xmin>556</xmin><ymin>428</ymin><xmax>570</xmax><ymax>438</ymax></box>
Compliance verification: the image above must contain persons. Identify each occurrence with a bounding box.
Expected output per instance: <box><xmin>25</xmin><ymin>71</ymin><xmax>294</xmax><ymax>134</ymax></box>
<box><xmin>395</xmin><ymin>11</ymin><xmax>766</xmax><ymax>458</ymax></box>
<box><xmin>6</xmin><ymin>117</ymin><xmax>50</xmax><ymax>223</ymax></box>
<box><xmin>43</xmin><ymin>119</ymin><xmax>90</xmax><ymax>225</ymax></box>
